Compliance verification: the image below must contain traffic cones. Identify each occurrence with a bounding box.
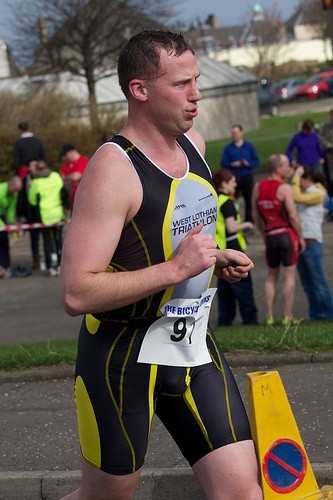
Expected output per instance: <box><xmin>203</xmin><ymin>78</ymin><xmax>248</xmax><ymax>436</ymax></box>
<box><xmin>244</xmin><ymin>369</ymin><xmax>333</xmax><ymax>500</ymax></box>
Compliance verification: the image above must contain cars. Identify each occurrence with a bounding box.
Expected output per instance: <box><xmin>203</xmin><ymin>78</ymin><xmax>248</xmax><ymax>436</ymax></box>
<box><xmin>259</xmin><ymin>71</ymin><xmax>333</xmax><ymax>118</ymax></box>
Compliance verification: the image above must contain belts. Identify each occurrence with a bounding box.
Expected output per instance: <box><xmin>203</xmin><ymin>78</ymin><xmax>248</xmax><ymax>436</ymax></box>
<box><xmin>304</xmin><ymin>239</ymin><xmax>316</xmax><ymax>244</ymax></box>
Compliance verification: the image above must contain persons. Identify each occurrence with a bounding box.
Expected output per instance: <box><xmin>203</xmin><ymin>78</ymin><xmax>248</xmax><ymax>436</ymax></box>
<box><xmin>0</xmin><ymin>110</ymin><xmax>333</xmax><ymax>328</ymax></box>
<box><xmin>59</xmin><ymin>30</ymin><xmax>263</xmax><ymax>500</ymax></box>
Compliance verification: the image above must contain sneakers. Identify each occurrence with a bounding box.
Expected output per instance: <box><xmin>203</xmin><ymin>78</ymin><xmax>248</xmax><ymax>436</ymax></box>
<box><xmin>282</xmin><ymin>315</ymin><xmax>300</xmax><ymax>326</ymax></box>
<box><xmin>265</xmin><ymin>315</ymin><xmax>274</xmax><ymax>326</ymax></box>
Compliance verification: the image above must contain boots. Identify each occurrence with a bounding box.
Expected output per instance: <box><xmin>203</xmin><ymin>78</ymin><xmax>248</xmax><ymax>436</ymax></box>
<box><xmin>33</xmin><ymin>255</ymin><xmax>40</xmax><ymax>270</ymax></box>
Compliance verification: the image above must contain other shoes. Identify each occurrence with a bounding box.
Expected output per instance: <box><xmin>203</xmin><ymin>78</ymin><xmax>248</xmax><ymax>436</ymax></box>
<box><xmin>0</xmin><ymin>266</ymin><xmax>12</xmax><ymax>278</ymax></box>
<box><xmin>49</xmin><ymin>268</ymin><xmax>56</xmax><ymax>277</ymax></box>
<box><xmin>57</xmin><ymin>266</ymin><xmax>61</xmax><ymax>275</ymax></box>
